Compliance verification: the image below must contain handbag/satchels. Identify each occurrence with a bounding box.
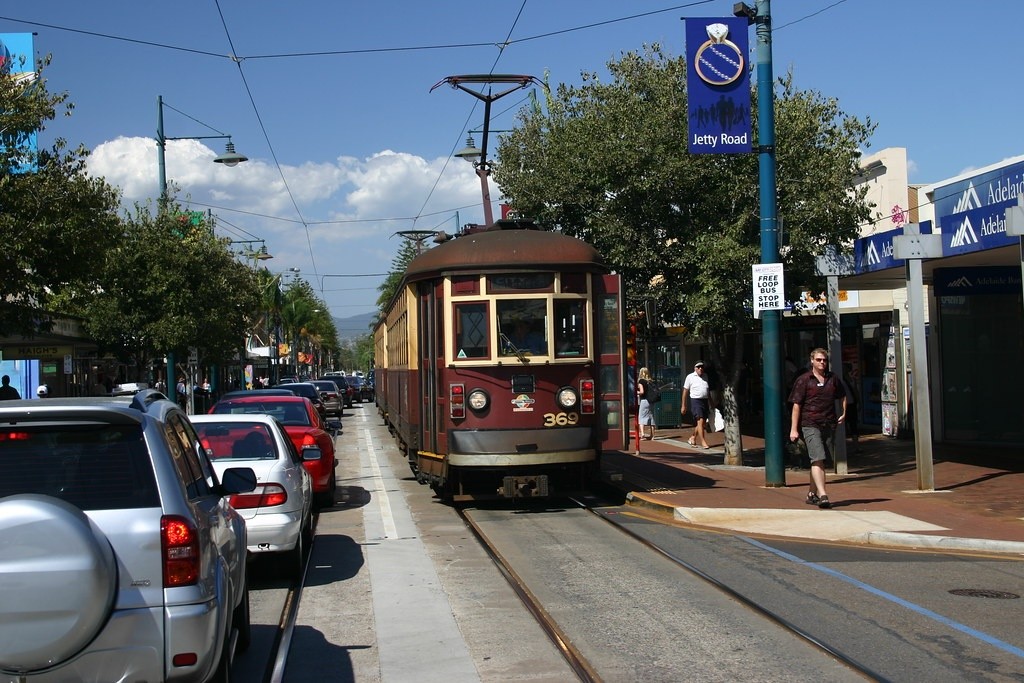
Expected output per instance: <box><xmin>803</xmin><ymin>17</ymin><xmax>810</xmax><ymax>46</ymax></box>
<box><xmin>708</xmin><ymin>408</ymin><xmax>725</xmax><ymax>432</ymax></box>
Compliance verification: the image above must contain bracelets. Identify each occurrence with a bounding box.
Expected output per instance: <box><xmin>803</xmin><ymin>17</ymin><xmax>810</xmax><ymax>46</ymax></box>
<box><xmin>571</xmin><ymin>342</ymin><xmax>573</xmax><ymax>347</ymax></box>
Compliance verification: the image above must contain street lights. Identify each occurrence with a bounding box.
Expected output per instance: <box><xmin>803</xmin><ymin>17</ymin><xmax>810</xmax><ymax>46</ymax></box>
<box><xmin>153</xmin><ymin>91</ymin><xmax>251</xmax><ymax>237</ymax></box>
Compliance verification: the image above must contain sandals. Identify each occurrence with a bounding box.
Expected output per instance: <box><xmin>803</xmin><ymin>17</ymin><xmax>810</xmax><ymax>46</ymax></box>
<box><xmin>819</xmin><ymin>495</ymin><xmax>830</xmax><ymax>507</ymax></box>
<box><xmin>805</xmin><ymin>493</ymin><xmax>820</xmax><ymax>504</ymax></box>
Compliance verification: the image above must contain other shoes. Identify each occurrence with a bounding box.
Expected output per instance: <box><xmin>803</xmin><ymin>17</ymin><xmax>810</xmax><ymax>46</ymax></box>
<box><xmin>702</xmin><ymin>444</ymin><xmax>709</xmax><ymax>449</ymax></box>
<box><xmin>640</xmin><ymin>434</ymin><xmax>656</xmax><ymax>442</ymax></box>
<box><xmin>688</xmin><ymin>440</ymin><xmax>698</xmax><ymax>447</ymax></box>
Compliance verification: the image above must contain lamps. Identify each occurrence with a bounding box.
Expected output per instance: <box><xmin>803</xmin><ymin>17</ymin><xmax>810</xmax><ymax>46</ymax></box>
<box><xmin>905</xmin><ymin>302</ymin><xmax>909</xmax><ymax>310</ymax></box>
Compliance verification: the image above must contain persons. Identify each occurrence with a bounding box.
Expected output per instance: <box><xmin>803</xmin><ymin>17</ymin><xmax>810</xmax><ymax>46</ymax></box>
<box><xmin>37</xmin><ymin>385</ymin><xmax>48</xmax><ymax>398</ymax></box>
<box><xmin>682</xmin><ymin>360</ymin><xmax>716</xmax><ymax>448</ymax></box>
<box><xmin>155</xmin><ymin>374</ymin><xmax>276</xmax><ymax>401</ymax></box>
<box><xmin>842</xmin><ymin>363</ymin><xmax>863</xmax><ymax>452</ymax></box>
<box><xmin>505</xmin><ymin>313</ymin><xmax>582</xmax><ymax>359</ymax></box>
<box><xmin>637</xmin><ymin>367</ymin><xmax>656</xmax><ymax>441</ymax></box>
<box><xmin>0</xmin><ymin>374</ymin><xmax>21</xmax><ymax>400</ymax></box>
<box><xmin>788</xmin><ymin>347</ymin><xmax>847</xmax><ymax>508</ymax></box>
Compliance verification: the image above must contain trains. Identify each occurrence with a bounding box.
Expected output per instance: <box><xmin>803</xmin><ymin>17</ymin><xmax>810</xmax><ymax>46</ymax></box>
<box><xmin>372</xmin><ymin>222</ymin><xmax>605</xmax><ymax>506</ymax></box>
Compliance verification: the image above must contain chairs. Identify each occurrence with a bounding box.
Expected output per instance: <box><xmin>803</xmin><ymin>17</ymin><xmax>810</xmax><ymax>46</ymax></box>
<box><xmin>286</xmin><ymin>410</ymin><xmax>304</xmax><ymax>421</ymax></box>
<box><xmin>232</xmin><ymin>440</ymin><xmax>258</xmax><ymax>459</ymax></box>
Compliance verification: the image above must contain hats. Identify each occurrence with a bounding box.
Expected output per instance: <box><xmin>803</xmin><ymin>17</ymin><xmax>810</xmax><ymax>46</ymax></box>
<box><xmin>179</xmin><ymin>378</ymin><xmax>185</xmax><ymax>382</ymax></box>
<box><xmin>37</xmin><ymin>386</ymin><xmax>47</xmax><ymax>394</ymax></box>
<box><xmin>694</xmin><ymin>360</ymin><xmax>704</xmax><ymax>366</ymax></box>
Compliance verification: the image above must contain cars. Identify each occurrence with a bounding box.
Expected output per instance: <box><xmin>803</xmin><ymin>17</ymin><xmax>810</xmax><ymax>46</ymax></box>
<box><xmin>1</xmin><ymin>383</ymin><xmax>248</xmax><ymax>683</ymax></box>
<box><xmin>228</xmin><ymin>373</ymin><xmax>370</xmax><ymax>433</ymax></box>
<box><xmin>141</xmin><ymin>420</ymin><xmax>313</xmax><ymax>569</ymax></box>
<box><xmin>194</xmin><ymin>398</ymin><xmax>337</xmax><ymax>505</ymax></box>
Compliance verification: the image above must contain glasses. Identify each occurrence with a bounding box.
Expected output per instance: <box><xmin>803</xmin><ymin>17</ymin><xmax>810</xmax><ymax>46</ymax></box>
<box><xmin>697</xmin><ymin>365</ymin><xmax>704</xmax><ymax>368</ymax></box>
<box><xmin>816</xmin><ymin>358</ymin><xmax>826</xmax><ymax>362</ymax></box>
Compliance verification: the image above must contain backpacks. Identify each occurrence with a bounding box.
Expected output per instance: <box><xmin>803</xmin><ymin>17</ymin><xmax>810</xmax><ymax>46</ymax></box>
<box><xmin>648</xmin><ymin>382</ymin><xmax>661</xmax><ymax>402</ymax></box>
<box><xmin>158</xmin><ymin>382</ymin><xmax>164</xmax><ymax>392</ymax></box>
